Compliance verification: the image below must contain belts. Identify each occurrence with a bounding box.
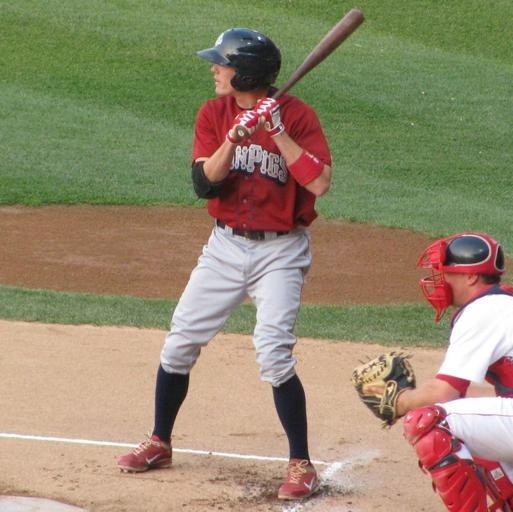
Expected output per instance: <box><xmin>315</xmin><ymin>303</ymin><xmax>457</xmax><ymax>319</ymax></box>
<box><xmin>216</xmin><ymin>219</ymin><xmax>291</xmax><ymax>238</ymax></box>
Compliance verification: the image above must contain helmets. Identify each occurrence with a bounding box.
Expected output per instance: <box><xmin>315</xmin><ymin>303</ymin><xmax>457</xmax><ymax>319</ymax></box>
<box><xmin>197</xmin><ymin>28</ymin><xmax>281</xmax><ymax>92</ymax></box>
<box><xmin>440</xmin><ymin>231</ymin><xmax>505</xmax><ymax>274</ymax></box>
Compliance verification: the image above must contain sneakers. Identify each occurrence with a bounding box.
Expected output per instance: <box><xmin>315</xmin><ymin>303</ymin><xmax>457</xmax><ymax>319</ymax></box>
<box><xmin>117</xmin><ymin>434</ymin><xmax>172</xmax><ymax>473</ymax></box>
<box><xmin>279</xmin><ymin>459</ymin><xmax>319</xmax><ymax>499</ymax></box>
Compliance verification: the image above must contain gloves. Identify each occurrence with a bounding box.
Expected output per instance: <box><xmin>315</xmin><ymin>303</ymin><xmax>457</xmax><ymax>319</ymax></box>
<box><xmin>254</xmin><ymin>96</ymin><xmax>285</xmax><ymax>137</ymax></box>
<box><xmin>226</xmin><ymin>110</ymin><xmax>260</xmax><ymax>143</ymax></box>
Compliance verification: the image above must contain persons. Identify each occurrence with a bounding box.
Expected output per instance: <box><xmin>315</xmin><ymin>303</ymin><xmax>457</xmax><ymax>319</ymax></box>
<box><xmin>349</xmin><ymin>232</ymin><xmax>513</xmax><ymax>511</ymax></box>
<box><xmin>114</xmin><ymin>27</ymin><xmax>336</xmax><ymax>501</ymax></box>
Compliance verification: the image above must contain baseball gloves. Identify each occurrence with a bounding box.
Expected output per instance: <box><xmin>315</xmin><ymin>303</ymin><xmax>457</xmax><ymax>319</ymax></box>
<box><xmin>353</xmin><ymin>347</ymin><xmax>417</xmax><ymax>427</ymax></box>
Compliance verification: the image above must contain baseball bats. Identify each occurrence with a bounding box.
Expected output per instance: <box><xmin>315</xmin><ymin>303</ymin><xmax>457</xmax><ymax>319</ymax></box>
<box><xmin>235</xmin><ymin>10</ymin><xmax>366</xmax><ymax>141</ymax></box>
